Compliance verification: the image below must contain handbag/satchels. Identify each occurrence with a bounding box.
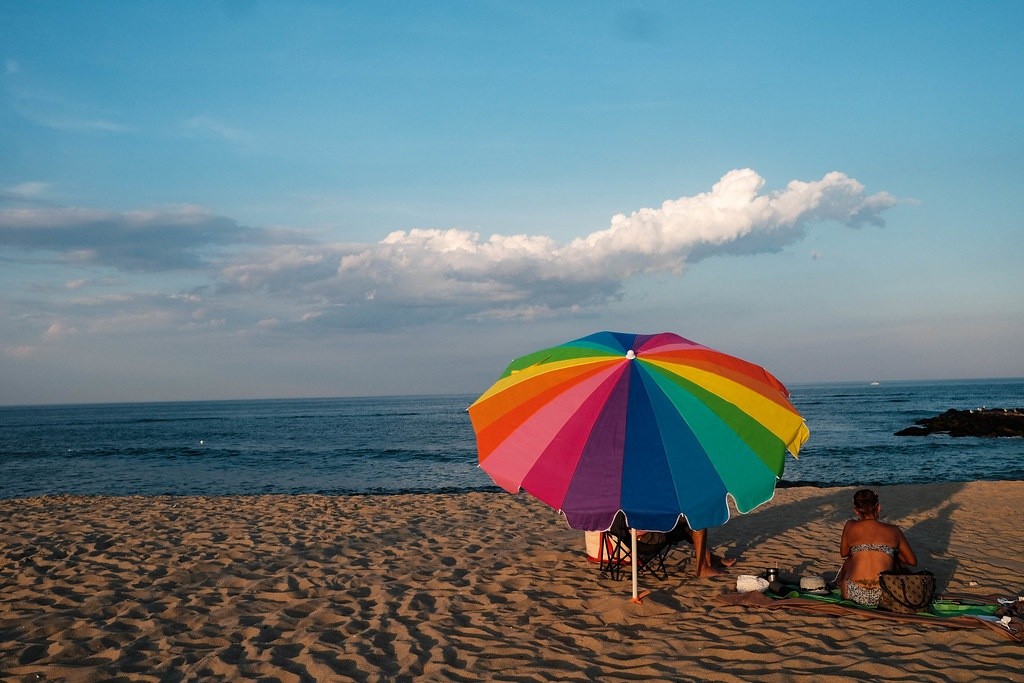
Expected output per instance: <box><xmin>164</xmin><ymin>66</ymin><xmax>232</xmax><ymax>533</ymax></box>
<box><xmin>877</xmin><ymin>570</ymin><xmax>935</xmax><ymax>615</ymax></box>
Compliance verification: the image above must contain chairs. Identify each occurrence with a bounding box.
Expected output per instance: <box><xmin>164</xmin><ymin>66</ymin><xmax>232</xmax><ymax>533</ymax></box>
<box><xmin>593</xmin><ymin>518</ymin><xmax>680</xmax><ymax>580</ymax></box>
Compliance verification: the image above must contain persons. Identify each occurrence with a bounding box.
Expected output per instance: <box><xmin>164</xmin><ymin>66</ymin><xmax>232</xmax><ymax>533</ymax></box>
<box><xmin>621</xmin><ymin>518</ymin><xmax>736</xmax><ymax>577</ymax></box>
<box><xmin>839</xmin><ymin>489</ymin><xmax>918</xmax><ymax>606</ymax></box>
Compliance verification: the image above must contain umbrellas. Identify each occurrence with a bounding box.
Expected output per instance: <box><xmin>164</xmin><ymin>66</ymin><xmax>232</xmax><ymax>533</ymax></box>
<box><xmin>466</xmin><ymin>331</ymin><xmax>811</xmax><ymax>600</ymax></box>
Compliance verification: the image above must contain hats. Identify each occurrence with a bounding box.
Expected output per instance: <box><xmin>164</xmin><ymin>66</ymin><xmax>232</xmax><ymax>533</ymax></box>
<box><xmin>797</xmin><ymin>576</ymin><xmax>833</xmax><ymax>597</ymax></box>
